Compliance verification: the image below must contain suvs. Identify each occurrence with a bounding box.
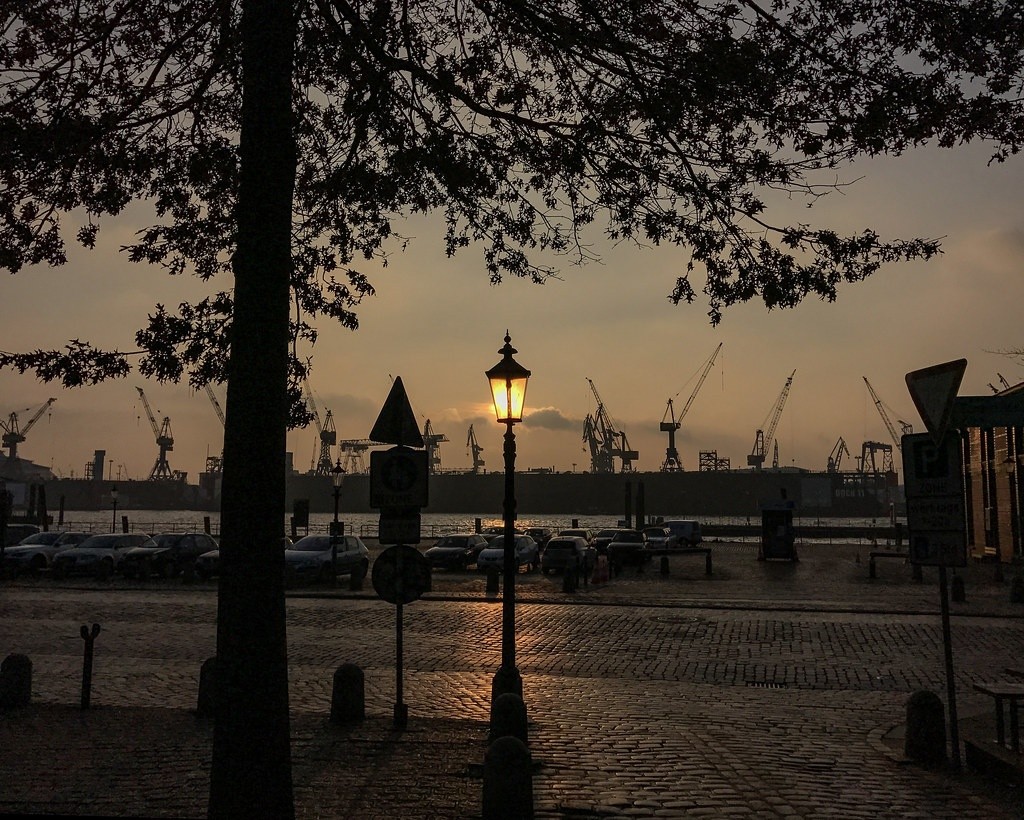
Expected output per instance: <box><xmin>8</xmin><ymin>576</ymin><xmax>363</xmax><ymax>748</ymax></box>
<box><xmin>51</xmin><ymin>531</ymin><xmax>158</xmax><ymax>582</ymax></box>
<box><xmin>116</xmin><ymin>530</ymin><xmax>219</xmax><ymax>584</ymax></box>
<box><xmin>0</xmin><ymin>530</ymin><xmax>99</xmax><ymax>577</ymax></box>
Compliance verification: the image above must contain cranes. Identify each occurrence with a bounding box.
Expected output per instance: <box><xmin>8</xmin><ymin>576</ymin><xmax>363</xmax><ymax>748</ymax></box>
<box><xmin>304</xmin><ymin>376</ymin><xmax>337</xmax><ymax>475</ymax></box>
<box><xmin>744</xmin><ymin>368</ymin><xmax>797</xmax><ymax>471</ymax></box>
<box><xmin>422</xmin><ymin>419</ymin><xmax>449</xmax><ymax>475</ymax></box>
<box><xmin>823</xmin><ymin>437</ymin><xmax>849</xmax><ymax>481</ymax></box>
<box><xmin>465</xmin><ymin>423</ymin><xmax>485</xmax><ymax>475</ymax></box>
<box><xmin>862</xmin><ymin>376</ymin><xmax>914</xmax><ymax>454</ymax></box>
<box><xmin>0</xmin><ymin>397</ymin><xmax>58</xmax><ymax>458</ymax></box>
<box><xmin>340</xmin><ymin>439</ymin><xmax>393</xmax><ymax>474</ymax></box>
<box><xmin>660</xmin><ymin>342</ymin><xmax>723</xmax><ymax>472</ymax></box>
<box><xmin>583</xmin><ymin>379</ymin><xmax>639</xmax><ymax>474</ymax></box>
<box><xmin>136</xmin><ymin>386</ymin><xmax>175</xmax><ymax>481</ymax></box>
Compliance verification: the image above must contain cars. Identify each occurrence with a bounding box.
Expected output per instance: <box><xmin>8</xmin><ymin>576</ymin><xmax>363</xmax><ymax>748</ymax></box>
<box><xmin>422</xmin><ymin>518</ymin><xmax>703</xmax><ymax>576</ymax></box>
<box><xmin>284</xmin><ymin>533</ymin><xmax>372</xmax><ymax>588</ymax></box>
<box><xmin>193</xmin><ymin>533</ymin><xmax>296</xmax><ymax>584</ymax></box>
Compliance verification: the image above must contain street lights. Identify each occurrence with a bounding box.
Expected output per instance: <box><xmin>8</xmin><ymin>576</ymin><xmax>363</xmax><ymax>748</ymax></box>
<box><xmin>330</xmin><ymin>457</ymin><xmax>347</xmax><ymax>588</ymax></box>
<box><xmin>482</xmin><ymin>325</ymin><xmax>533</xmax><ymax>744</ymax></box>
<box><xmin>110</xmin><ymin>484</ymin><xmax>120</xmax><ymax>534</ymax></box>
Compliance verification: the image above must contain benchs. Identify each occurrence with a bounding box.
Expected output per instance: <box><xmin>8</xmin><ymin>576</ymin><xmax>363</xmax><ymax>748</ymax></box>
<box><xmin>614</xmin><ymin>548</ymin><xmax>712</xmax><ymax>576</ymax></box>
<box><xmin>869</xmin><ymin>553</ymin><xmax>922</xmax><ymax>583</ymax></box>
<box><xmin>973</xmin><ymin>669</ymin><xmax>1024</xmax><ymax>755</ymax></box>
